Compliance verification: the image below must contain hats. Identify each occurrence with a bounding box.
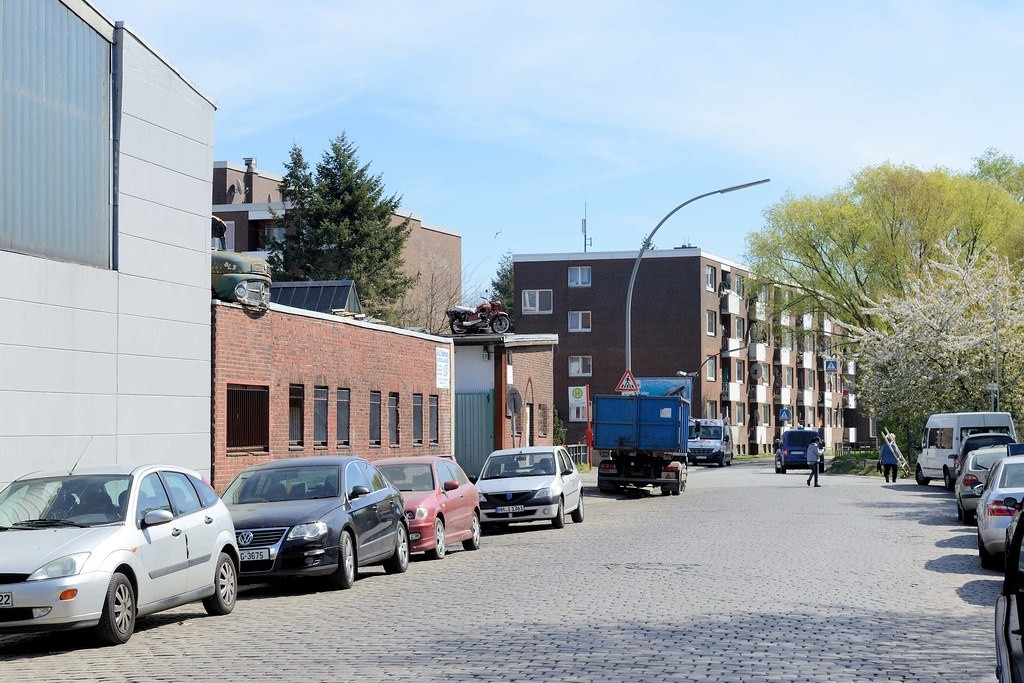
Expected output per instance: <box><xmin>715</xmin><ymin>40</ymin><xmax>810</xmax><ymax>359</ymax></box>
<box><xmin>815</xmin><ymin>436</ymin><xmax>822</xmax><ymax>444</ymax></box>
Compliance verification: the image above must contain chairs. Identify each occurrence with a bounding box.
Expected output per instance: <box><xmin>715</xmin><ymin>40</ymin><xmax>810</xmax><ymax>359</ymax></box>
<box><xmin>318</xmin><ymin>475</ymin><xmax>338</xmax><ymax>495</ymax></box>
<box><xmin>387</xmin><ymin>469</ymin><xmax>407</xmax><ymax>489</ymax></box>
<box><xmin>169</xmin><ymin>487</ymin><xmax>187</xmax><ymax>514</ymax></box>
<box><xmin>502</xmin><ymin>462</ymin><xmax>519</xmax><ymax>475</ymax></box>
<box><xmin>539</xmin><ymin>459</ymin><xmax>554</xmax><ymax>474</ymax></box>
<box><xmin>289</xmin><ymin>482</ymin><xmax>307</xmax><ymax>499</ymax></box>
<box><xmin>712</xmin><ymin>430</ymin><xmax>720</xmax><ymax>438</ymax></box>
<box><xmin>117</xmin><ymin>488</ymin><xmax>148</xmax><ymax>521</ymax></box>
<box><xmin>85</xmin><ymin>492</ymin><xmax>114</xmax><ymax>517</ymax></box>
<box><xmin>274</xmin><ymin>484</ymin><xmax>287</xmax><ymax>501</ymax></box>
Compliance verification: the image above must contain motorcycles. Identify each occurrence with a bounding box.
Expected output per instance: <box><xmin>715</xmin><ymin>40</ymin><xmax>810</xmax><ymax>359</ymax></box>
<box><xmin>445</xmin><ymin>289</ymin><xmax>511</xmax><ymax>335</ymax></box>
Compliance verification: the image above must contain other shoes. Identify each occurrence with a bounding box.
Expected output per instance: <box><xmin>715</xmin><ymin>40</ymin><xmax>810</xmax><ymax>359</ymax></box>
<box><xmin>807</xmin><ymin>479</ymin><xmax>810</xmax><ymax>486</ymax></box>
<box><xmin>886</xmin><ymin>480</ymin><xmax>889</xmax><ymax>482</ymax></box>
<box><xmin>814</xmin><ymin>484</ymin><xmax>822</xmax><ymax>487</ymax></box>
<box><xmin>893</xmin><ymin>479</ymin><xmax>896</xmax><ymax>482</ymax></box>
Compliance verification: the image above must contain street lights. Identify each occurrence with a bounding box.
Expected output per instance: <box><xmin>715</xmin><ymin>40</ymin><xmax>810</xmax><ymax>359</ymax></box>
<box><xmin>624</xmin><ymin>176</ymin><xmax>774</xmax><ymax>376</ymax></box>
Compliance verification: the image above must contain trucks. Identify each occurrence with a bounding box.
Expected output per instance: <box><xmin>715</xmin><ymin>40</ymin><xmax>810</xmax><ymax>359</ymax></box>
<box><xmin>589</xmin><ymin>375</ymin><xmax>702</xmax><ymax>496</ymax></box>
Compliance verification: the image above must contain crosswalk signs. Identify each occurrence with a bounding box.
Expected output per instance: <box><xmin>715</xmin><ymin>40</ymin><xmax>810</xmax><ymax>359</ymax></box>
<box><xmin>779</xmin><ymin>409</ymin><xmax>790</xmax><ymax>422</ymax></box>
<box><xmin>614</xmin><ymin>367</ymin><xmax>639</xmax><ymax>392</ymax></box>
<box><xmin>825</xmin><ymin>361</ymin><xmax>838</xmax><ymax>372</ymax></box>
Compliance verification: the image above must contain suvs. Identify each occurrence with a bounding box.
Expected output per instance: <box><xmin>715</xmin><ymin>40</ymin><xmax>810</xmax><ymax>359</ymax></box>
<box><xmin>946</xmin><ymin>431</ymin><xmax>1017</xmax><ymax>483</ymax></box>
<box><xmin>774</xmin><ymin>429</ymin><xmax>825</xmax><ymax>474</ymax></box>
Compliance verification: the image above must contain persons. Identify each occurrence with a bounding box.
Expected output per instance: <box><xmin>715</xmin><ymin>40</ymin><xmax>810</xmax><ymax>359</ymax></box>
<box><xmin>879</xmin><ymin>433</ymin><xmax>900</xmax><ymax>482</ymax></box>
<box><xmin>807</xmin><ymin>436</ymin><xmax>825</xmax><ymax>487</ymax></box>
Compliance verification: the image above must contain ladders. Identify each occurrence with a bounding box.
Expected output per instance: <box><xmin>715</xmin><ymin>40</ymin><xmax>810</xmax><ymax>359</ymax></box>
<box><xmin>881</xmin><ymin>427</ymin><xmax>912</xmax><ymax>476</ymax></box>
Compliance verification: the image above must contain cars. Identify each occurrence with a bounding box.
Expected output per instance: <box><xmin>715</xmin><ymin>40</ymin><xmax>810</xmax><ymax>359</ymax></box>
<box><xmin>954</xmin><ymin>448</ymin><xmax>1007</xmax><ymax>526</ymax></box>
<box><xmin>368</xmin><ymin>453</ymin><xmax>482</xmax><ymax>561</ymax></box>
<box><xmin>468</xmin><ymin>445</ymin><xmax>585</xmax><ymax>535</ymax></box>
<box><xmin>973</xmin><ymin>455</ymin><xmax>1024</xmax><ymax>558</ymax></box>
<box><xmin>217</xmin><ymin>454</ymin><xmax>411</xmax><ymax>589</ymax></box>
<box><xmin>0</xmin><ymin>464</ymin><xmax>242</xmax><ymax>644</ymax></box>
<box><xmin>993</xmin><ymin>496</ymin><xmax>1024</xmax><ymax>683</ymax></box>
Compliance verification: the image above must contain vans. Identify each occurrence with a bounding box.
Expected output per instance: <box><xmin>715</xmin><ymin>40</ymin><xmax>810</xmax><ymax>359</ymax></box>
<box><xmin>687</xmin><ymin>417</ymin><xmax>734</xmax><ymax>467</ymax></box>
<box><xmin>915</xmin><ymin>411</ymin><xmax>1018</xmax><ymax>492</ymax></box>
